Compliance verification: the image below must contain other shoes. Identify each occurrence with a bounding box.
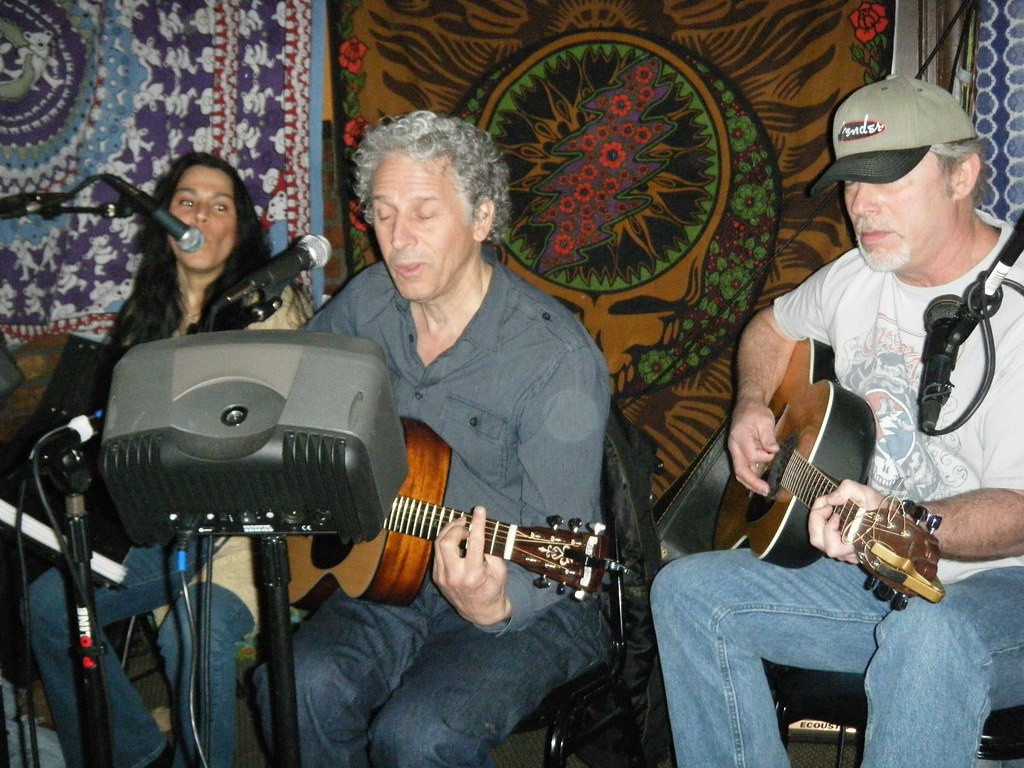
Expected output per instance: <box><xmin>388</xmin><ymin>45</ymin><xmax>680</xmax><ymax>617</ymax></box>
<box><xmin>143</xmin><ymin>743</ymin><xmax>174</xmax><ymax>768</ymax></box>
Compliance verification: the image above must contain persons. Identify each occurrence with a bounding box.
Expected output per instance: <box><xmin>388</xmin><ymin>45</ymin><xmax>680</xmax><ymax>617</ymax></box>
<box><xmin>652</xmin><ymin>76</ymin><xmax>1023</xmax><ymax>768</ymax></box>
<box><xmin>249</xmin><ymin>108</ymin><xmax>615</xmax><ymax>768</ymax></box>
<box><xmin>18</xmin><ymin>153</ymin><xmax>314</xmax><ymax>768</ymax></box>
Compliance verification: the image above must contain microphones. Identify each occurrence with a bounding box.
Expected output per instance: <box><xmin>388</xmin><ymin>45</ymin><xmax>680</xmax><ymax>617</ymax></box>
<box><xmin>218</xmin><ymin>234</ymin><xmax>332</xmax><ymax>305</ymax></box>
<box><xmin>99</xmin><ymin>174</ymin><xmax>204</xmax><ymax>253</ymax></box>
<box><xmin>918</xmin><ymin>294</ymin><xmax>966</xmax><ymax>432</ymax></box>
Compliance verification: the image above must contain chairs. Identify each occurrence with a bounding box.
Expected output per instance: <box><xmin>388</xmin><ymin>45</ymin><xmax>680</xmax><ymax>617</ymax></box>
<box><xmin>514</xmin><ymin>410</ymin><xmax>659</xmax><ymax>767</ymax></box>
<box><xmin>764</xmin><ymin>662</ymin><xmax>1024</xmax><ymax>767</ymax></box>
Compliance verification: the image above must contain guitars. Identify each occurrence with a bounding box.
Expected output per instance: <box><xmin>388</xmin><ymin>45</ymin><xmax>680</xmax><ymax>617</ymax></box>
<box><xmin>709</xmin><ymin>336</ymin><xmax>949</xmax><ymax>609</ymax></box>
<box><xmin>290</xmin><ymin>413</ymin><xmax>630</xmax><ymax>612</ymax></box>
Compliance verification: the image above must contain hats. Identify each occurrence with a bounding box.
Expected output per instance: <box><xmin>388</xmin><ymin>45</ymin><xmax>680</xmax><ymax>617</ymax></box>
<box><xmin>810</xmin><ymin>74</ymin><xmax>976</xmax><ymax>207</ymax></box>
